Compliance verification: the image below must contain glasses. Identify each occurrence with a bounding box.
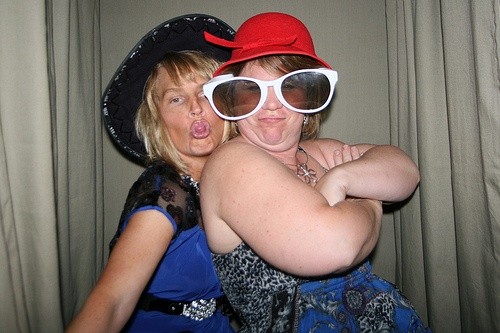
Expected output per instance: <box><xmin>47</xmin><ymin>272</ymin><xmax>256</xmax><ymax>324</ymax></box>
<box><xmin>203</xmin><ymin>68</ymin><xmax>338</xmax><ymax>120</ymax></box>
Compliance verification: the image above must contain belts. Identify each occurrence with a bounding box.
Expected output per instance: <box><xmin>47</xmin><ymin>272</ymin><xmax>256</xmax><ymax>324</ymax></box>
<box><xmin>136</xmin><ymin>290</ymin><xmax>228</xmax><ymax>322</ymax></box>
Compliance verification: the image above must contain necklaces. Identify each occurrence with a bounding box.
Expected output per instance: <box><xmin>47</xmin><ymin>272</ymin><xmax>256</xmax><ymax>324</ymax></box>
<box><xmin>181</xmin><ymin>172</ymin><xmax>202</xmax><ymax>197</ymax></box>
<box><xmin>284</xmin><ymin>145</ymin><xmax>320</xmax><ymax>185</ymax></box>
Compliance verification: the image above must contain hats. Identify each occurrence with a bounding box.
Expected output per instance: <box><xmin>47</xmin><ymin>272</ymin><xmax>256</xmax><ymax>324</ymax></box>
<box><xmin>101</xmin><ymin>14</ymin><xmax>236</xmax><ymax>167</ymax></box>
<box><xmin>203</xmin><ymin>12</ymin><xmax>333</xmax><ymax>77</ymax></box>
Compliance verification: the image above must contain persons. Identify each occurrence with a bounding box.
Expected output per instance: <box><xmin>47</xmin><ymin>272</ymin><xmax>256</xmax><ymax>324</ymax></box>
<box><xmin>63</xmin><ymin>48</ymin><xmax>242</xmax><ymax>333</ymax></box>
<box><xmin>198</xmin><ymin>12</ymin><xmax>434</xmax><ymax>333</ymax></box>
